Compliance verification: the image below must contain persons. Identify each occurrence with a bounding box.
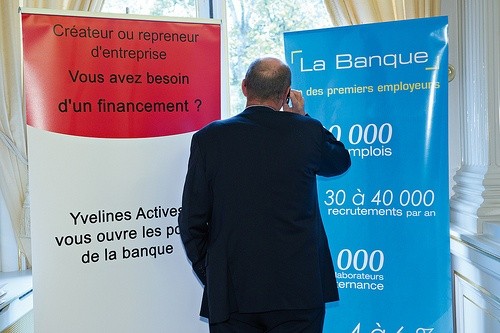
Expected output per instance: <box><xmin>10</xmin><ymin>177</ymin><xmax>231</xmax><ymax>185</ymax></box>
<box><xmin>176</xmin><ymin>55</ymin><xmax>351</xmax><ymax>333</ymax></box>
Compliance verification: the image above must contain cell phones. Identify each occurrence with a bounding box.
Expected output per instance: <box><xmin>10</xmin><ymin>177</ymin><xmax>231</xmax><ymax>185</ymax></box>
<box><xmin>286</xmin><ymin>88</ymin><xmax>291</xmax><ymax>104</ymax></box>
